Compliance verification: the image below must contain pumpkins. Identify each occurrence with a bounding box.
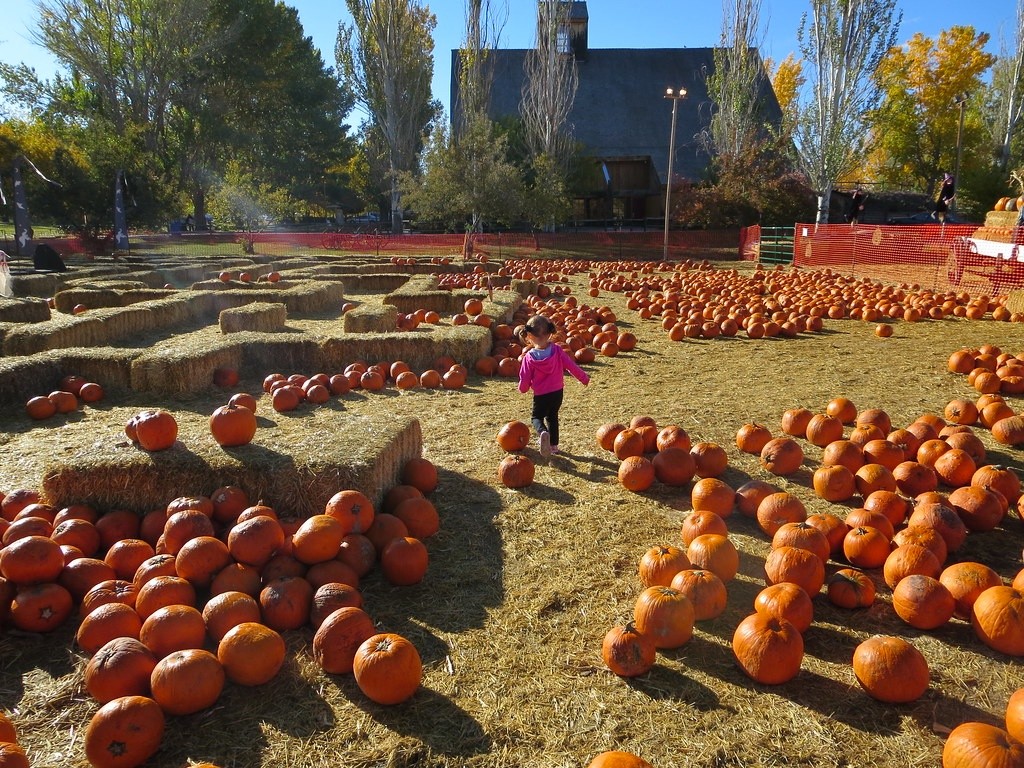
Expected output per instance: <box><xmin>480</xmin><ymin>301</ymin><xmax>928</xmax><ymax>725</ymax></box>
<box><xmin>26</xmin><ymin>252</ymin><xmax>1024</xmax><ymax>487</ymax></box>
<box><xmin>587</xmin><ymin>477</ymin><xmax>1024</xmax><ymax>768</ymax></box>
<box><xmin>978</xmin><ymin>194</ymin><xmax>1024</xmax><ymax>235</ymax></box>
<box><xmin>0</xmin><ymin>477</ymin><xmax>439</xmax><ymax>768</ymax></box>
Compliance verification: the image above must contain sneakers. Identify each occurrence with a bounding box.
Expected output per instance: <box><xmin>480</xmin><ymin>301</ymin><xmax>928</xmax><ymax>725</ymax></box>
<box><xmin>540</xmin><ymin>431</ymin><xmax>551</xmax><ymax>458</ymax></box>
<box><xmin>550</xmin><ymin>446</ymin><xmax>558</xmax><ymax>453</ymax></box>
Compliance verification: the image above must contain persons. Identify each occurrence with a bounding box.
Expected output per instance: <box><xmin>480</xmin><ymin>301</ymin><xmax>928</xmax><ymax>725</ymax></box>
<box><xmin>613</xmin><ymin>216</ymin><xmax>623</xmax><ymax>231</ymax></box>
<box><xmin>185</xmin><ymin>214</ymin><xmax>195</xmax><ymax>232</ymax></box>
<box><xmin>844</xmin><ymin>189</ymin><xmax>865</xmax><ymax>224</ymax></box>
<box><xmin>518</xmin><ymin>316</ymin><xmax>590</xmax><ymax>457</ymax></box>
<box><xmin>935</xmin><ymin>176</ymin><xmax>954</xmax><ymax>222</ymax></box>
